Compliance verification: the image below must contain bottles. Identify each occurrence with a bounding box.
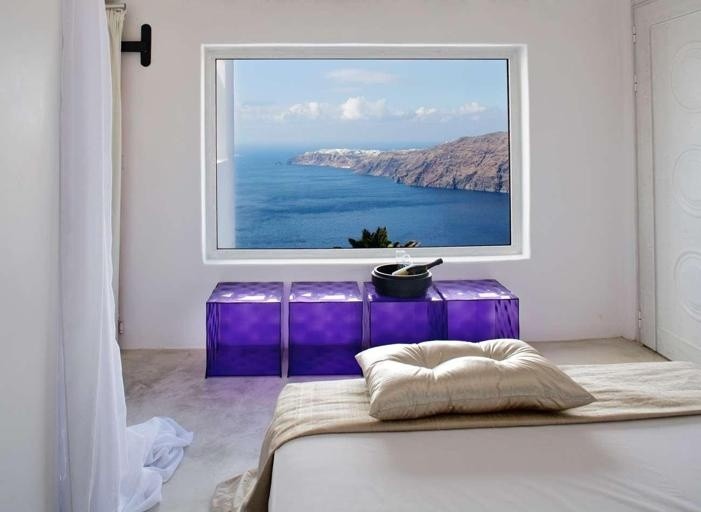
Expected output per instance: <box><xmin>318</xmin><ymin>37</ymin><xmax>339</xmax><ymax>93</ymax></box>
<box><xmin>392</xmin><ymin>258</ymin><xmax>443</xmax><ymax>275</ymax></box>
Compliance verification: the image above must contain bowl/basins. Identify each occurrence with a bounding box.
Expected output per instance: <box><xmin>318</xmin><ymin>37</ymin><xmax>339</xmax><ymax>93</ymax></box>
<box><xmin>371</xmin><ymin>264</ymin><xmax>432</xmax><ymax>298</ymax></box>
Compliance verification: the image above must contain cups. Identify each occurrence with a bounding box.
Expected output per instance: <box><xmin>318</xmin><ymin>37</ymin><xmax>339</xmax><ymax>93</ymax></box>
<box><xmin>395</xmin><ymin>250</ymin><xmax>405</xmax><ymax>263</ymax></box>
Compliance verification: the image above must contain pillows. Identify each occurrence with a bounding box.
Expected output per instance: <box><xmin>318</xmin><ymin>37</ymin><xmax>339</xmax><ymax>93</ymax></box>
<box><xmin>352</xmin><ymin>335</ymin><xmax>600</xmax><ymax>422</ymax></box>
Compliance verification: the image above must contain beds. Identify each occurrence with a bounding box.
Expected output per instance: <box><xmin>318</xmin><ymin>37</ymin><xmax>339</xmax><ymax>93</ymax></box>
<box><xmin>262</xmin><ymin>357</ymin><xmax>699</xmax><ymax>511</ymax></box>
